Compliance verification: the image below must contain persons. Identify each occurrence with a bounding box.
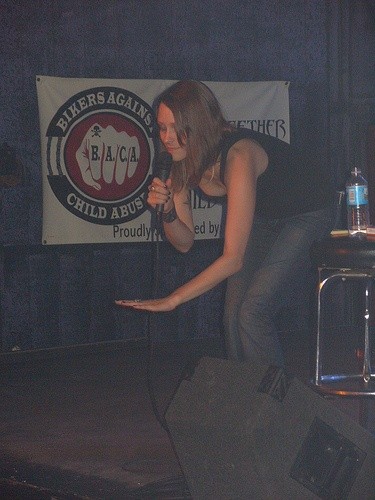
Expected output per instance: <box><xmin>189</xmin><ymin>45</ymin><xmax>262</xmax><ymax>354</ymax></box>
<box><xmin>114</xmin><ymin>80</ymin><xmax>336</xmax><ymax>369</ymax></box>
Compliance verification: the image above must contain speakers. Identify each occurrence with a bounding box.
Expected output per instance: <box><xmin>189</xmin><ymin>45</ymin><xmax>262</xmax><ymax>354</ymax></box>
<box><xmin>165</xmin><ymin>357</ymin><xmax>375</xmax><ymax>500</ymax></box>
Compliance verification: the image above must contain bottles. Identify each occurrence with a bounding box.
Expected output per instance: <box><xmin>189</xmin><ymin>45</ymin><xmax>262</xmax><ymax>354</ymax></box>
<box><xmin>346</xmin><ymin>167</ymin><xmax>370</xmax><ymax>241</ymax></box>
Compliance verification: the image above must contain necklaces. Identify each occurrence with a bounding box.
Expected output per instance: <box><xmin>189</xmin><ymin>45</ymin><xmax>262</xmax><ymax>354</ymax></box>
<box><xmin>207</xmin><ymin>167</ymin><xmax>215</xmax><ymax>184</ymax></box>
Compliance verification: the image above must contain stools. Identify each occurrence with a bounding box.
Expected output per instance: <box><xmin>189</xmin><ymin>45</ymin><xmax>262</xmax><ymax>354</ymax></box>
<box><xmin>311</xmin><ymin>238</ymin><xmax>375</xmax><ymax>397</ymax></box>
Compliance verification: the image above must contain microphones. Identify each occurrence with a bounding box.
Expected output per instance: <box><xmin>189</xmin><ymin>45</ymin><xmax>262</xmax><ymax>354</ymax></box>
<box><xmin>155</xmin><ymin>151</ymin><xmax>173</xmax><ymax>222</ymax></box>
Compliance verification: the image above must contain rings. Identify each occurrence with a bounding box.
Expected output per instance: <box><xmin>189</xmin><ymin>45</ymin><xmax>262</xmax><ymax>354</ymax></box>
<box><xmin>150</xmin><ymin>186</ymin><xmax>155</xmax><ymax>192</ymax></box>
